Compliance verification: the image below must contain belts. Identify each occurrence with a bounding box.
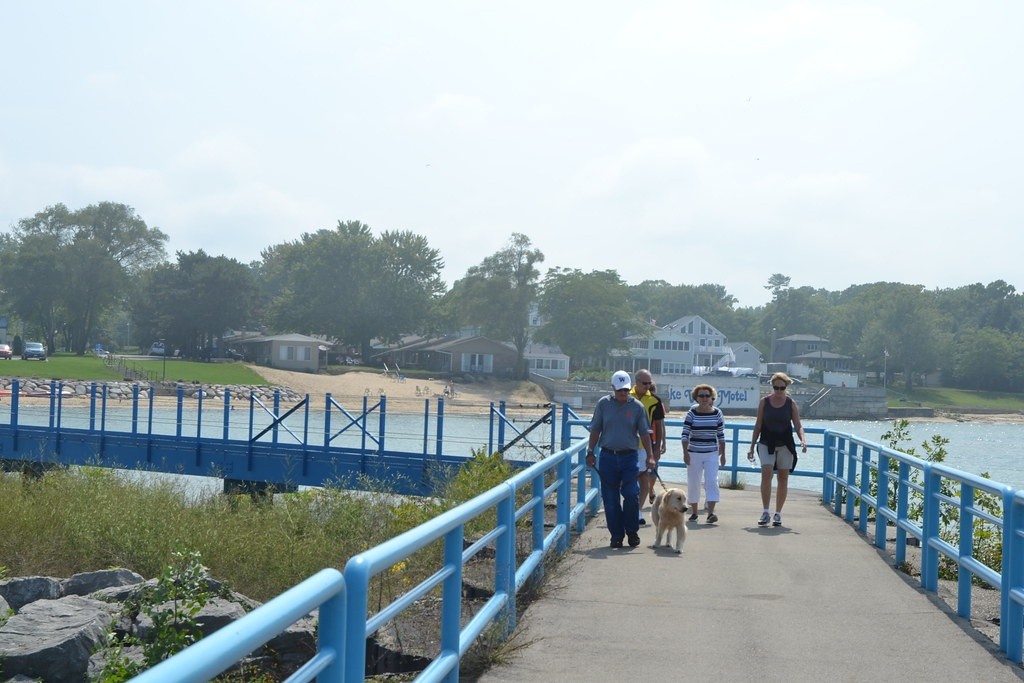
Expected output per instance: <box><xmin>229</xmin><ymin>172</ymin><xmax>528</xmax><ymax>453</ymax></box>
<box><xmin>602</xmin><ymin>447</ymin><xmax>634</xmax><ymax>455</ymax></box>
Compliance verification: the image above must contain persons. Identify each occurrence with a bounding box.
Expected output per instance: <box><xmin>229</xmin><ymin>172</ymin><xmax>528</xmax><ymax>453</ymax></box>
<box><xmin>336</xmin><ymin>354</ymin><xmax>345</xmax><ymax>365</ymax></box>
<box><xmin>625</xmin><ymin>368</ymin><xmax>665</xmax><ymax>530</ymax></box>
<box><xmin>688</xmin><ymin>388</ymin><xmax>722</xmax><ymax>509</ymax></box>
<box><xmin>638</xmin><ymin>379</ymin><xmax>667</xmax><ymax>505</ymax></box>
<box><xmin>585</xmin><ymin>369</ymin><xmax>656</xmax><ymax>548</ymax></box>
<box><xmin>681</xmin><ymin>384</ymin><xmax>726</xmax><ymax>524</ymax></box>
<box><xmin>449</xmin><ymin>379</ymin><xmax>455</xmax><ymax>398</ymax></box>
<box><xmin>444</xmin><ymin>385</ymin><xmax>450</xmax><ymax>396</ymax></box>
<box><xmin>747</xmin><ymin>371</ymin><xmax>808</xmax><ymax>526</ymax></box>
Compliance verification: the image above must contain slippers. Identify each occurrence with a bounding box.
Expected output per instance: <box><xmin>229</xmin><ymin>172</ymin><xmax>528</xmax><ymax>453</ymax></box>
<box><xmin>649</xmin><ymin>494</ymin><xmax>655</xmax><ymax>504</ymax></box>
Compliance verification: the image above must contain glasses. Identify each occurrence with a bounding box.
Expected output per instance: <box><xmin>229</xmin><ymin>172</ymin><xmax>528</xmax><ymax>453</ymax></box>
<box><xmin>773</xmin><ymin>386</ymin><xmax>786</xmax><ymax>390</ymax></box>
<box><xmin>639</xmin><ymin>381</ymin><xmax>651</xmax><ymax>385</ymax></box>
<box><xmin>696</xmin><ymin>394</ymin><xmax>712</xmax><ymax>398</ymax></box>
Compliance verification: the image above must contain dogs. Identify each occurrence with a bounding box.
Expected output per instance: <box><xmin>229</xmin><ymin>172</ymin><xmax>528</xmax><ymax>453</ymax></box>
<box><xmin>651</xmin><ymin>488</ymin><xmax>689</xmax><ymax>553</ymax></box>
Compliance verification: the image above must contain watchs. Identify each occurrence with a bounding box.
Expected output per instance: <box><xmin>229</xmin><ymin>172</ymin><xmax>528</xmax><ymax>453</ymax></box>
<box><xmin>586</xmin><ymin>447</ymin><xmax>593</xmax><ymax>454</ymax></box>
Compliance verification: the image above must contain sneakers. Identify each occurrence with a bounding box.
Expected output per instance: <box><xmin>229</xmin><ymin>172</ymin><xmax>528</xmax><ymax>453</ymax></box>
<box><xmin>757</xmin><ymin>512</ymin><xmax>770</xmax><ymax>525</ymax></box>
<box><xmin>689</xmin><ymin>514</ymin><xmax>698</xmax><ymax>521</ymax></box>
<box><xmin>706</xmin><ymin>514</ymin><xmax>718</xmax><ymax>523</ymax></box>
<box><xmin>772</xmin><ymin>514</ymin><xmax>781</xmax><ymax>526</ymax></box>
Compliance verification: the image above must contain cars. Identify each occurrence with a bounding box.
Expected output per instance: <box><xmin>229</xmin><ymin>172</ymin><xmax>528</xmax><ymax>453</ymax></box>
<box><xmin>0</xmin><ymin>343</ymin><xmax>14</xmax><ymax>359</ymax></box>
<box><xmin>91</xmin><ymin>348</ymin><xmax>110</xmax><ymax>355</ymax></box>
<box><xmin>22</xmin><ymin>342</ymin><xmax>48</xmax><ymax>360</ymax></box>
<box><xmin>204</xmin><ymin>347</ymin><xmax>245</xmax><ymax>360</ymax></box>
<box><xmin>147</xmin><ymin>341</ymin><xmax>165</xmax><ymax>355</ymax></box>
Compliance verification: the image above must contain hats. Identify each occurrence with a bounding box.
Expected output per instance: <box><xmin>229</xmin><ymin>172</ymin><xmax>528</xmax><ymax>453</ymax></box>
<box><xmin>611</xmin><ymin>370</ymin><xmax>632</xmax><ymax>390</ymax></box>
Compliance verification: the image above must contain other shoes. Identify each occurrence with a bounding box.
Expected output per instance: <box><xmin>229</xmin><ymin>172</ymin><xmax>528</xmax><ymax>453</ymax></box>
<box><xmin>626</xmin><ymin>532</ymin><xmax>640</xmax><ymax>546</ymax></box>
<box><xmin>611</xmin><ymin>540</ymin><xmax>622</xmax><ymax>547</ymax></box>
<box><xmin>639</xmin><ymin>510</ymin><xmax>646</xmax><ymax>527</ymax></box>
